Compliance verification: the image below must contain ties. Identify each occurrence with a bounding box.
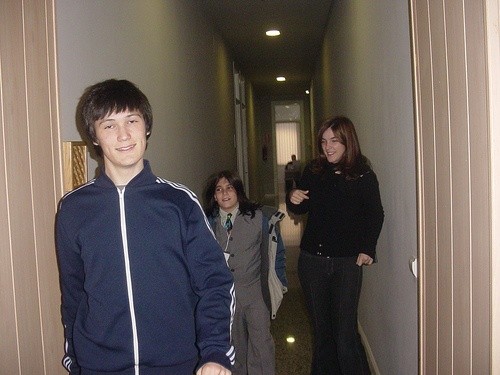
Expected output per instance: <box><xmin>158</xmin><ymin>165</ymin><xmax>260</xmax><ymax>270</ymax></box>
<box><xmin>225</xmin><ymin>214</ymin><xmax>233</xmax><ymax>233</ymax></box>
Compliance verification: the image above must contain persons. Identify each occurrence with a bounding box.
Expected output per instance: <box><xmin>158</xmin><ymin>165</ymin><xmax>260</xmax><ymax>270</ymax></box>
<box><xmin>285</xmin><ymin>154</ymin><xmax>301</xmax><ymax>171</ymax></box>
<box><xmin>54</xmin><ymin>79</ymin><xmax>236</xmax><ymax>375</ymax></box>
<box><xmin>202</xmin><ymin>170</ymin><xmax>288</xmax><ymax>375</ymax></box>
<box><xmin>285</xmin><ymin>115</ymin><xmax>385</xmax><ymax>375</ymax></box>
